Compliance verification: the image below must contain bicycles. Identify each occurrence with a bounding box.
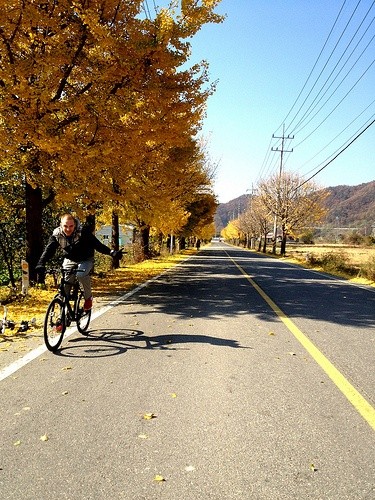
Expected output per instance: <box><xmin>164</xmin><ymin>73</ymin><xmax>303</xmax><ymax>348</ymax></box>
<box><xmin>42</xmin><ymin>267</ymin><xmax>92</xmax><ymax>352</ymax></box>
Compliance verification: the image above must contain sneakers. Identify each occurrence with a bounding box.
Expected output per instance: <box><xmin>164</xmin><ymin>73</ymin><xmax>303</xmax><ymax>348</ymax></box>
<box><xmin>83</xmin><ymin>293</ymin><xmax>93</xmax><ymax>311</ymax></box>
<box><xmin>55</xmin><ymin>316</ymin><xmax>62</xmax><ymax>331</ymax></box>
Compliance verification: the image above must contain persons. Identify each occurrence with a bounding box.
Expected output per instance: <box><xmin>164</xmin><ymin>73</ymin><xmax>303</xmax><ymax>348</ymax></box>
<box><xmin>35</xmin><ymin>213</ymin><xmax>129</xmax><ymax>333</ymax></box>
<box><xmin>195</xmin><ymin>239</ymin><xmax>202</xmax><ymax>251</ymax></box>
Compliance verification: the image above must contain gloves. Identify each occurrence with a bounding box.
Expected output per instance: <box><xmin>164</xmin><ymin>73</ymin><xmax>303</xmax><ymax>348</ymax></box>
<box><xmin>36</xmin><ymin>264</ymin><xmax>46</xmax><ymax>275</ymax></box>
<box><xmin>111</xmin><ymin>247</ymin><xmax>129</xmax><ymax>261</ymax></box>
